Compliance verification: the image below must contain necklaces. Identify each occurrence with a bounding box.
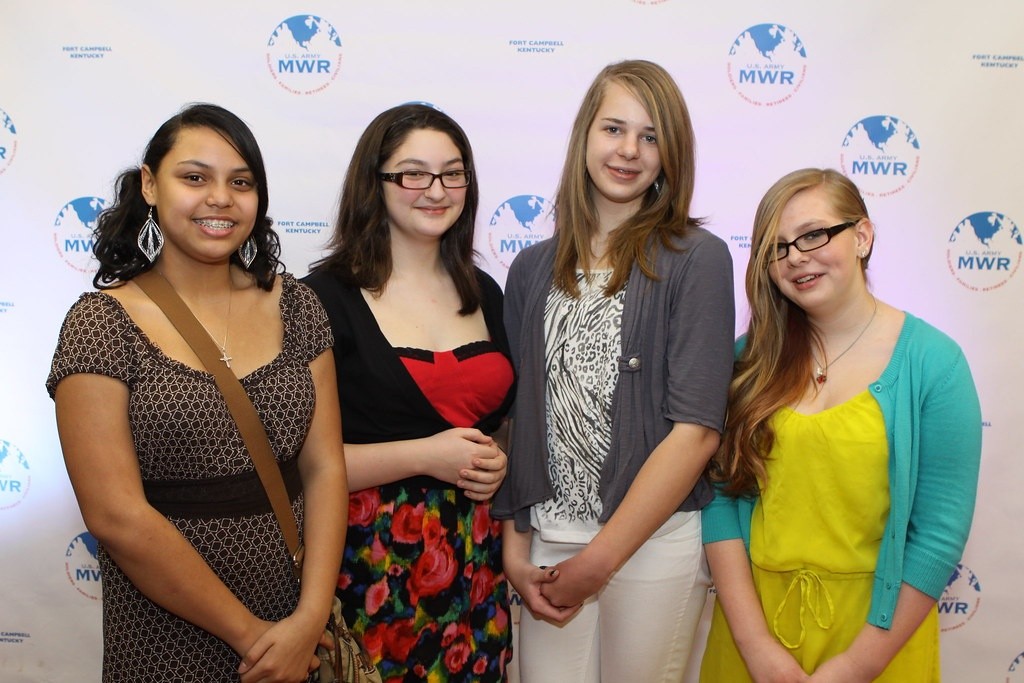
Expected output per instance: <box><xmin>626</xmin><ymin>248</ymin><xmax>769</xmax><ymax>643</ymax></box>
<box><xmin>812</xmin><ymin>293</ymin><xmax>877</xmax><ymax>384</ymax></box>
<box><xmin>159</xmin><ymin>270</ymin><xmax>232</xmax><ymax>369</ymax></box>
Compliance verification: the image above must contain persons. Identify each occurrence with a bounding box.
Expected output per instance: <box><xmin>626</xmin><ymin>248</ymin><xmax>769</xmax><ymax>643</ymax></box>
<box><xmin>292</xmin><ymin>105</ymin><xmax>519</xmax><ymax>683</ymax></box>
<box><xmin>45</xmin><ymin>110</ymin><xmax>352</xmax><ymax>683</ymax></box>
<box><xmin>701</xmin><ymin>167</ymin><xmax>984</xmax><ymax>683</ymax></box>
<box><xmin>504</xmin><ymin>58</ymin><xmax>736</xmax><ymax>683</ymax></box>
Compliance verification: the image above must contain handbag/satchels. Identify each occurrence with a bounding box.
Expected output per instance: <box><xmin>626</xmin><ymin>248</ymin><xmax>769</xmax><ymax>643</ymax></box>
<box><xmin>318</xmin><ymin>596</ymin><xmax>383</xmax><ymax>683</ymax></box>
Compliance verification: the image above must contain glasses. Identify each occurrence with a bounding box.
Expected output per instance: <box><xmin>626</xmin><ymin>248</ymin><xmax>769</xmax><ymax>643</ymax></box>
<box><xmin>766</xmin><ymin>221</ymin><xmax>858</xmax><ymax>263</ymax></box>
<box><xmin>379</xmin><ymin>169</ymin><xmax>472</xmax><ymax>190</ymax></box>
